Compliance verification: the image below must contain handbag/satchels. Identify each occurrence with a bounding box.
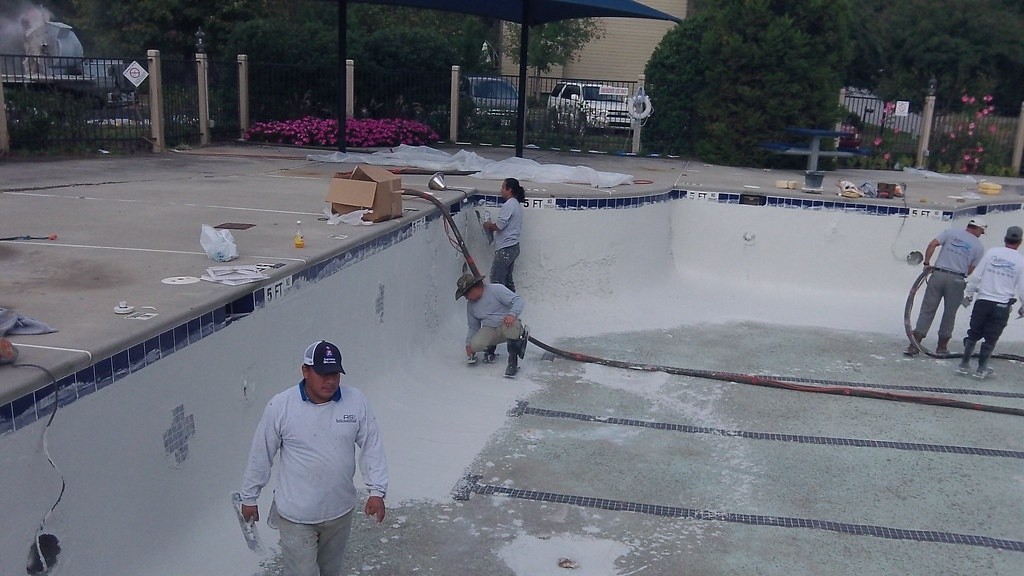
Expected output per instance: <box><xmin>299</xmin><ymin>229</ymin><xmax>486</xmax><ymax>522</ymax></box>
<box><xmin>199</xmin><ymin>223</ymin><xmax>240</xmax><ymax>262</ymax></box>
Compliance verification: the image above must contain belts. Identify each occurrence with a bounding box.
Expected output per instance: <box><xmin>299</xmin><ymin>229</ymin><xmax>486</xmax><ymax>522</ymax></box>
<box><xmin>934</xmin><ymin>268</ymin><xmax>964</xmax><ymax>276</ymax></box>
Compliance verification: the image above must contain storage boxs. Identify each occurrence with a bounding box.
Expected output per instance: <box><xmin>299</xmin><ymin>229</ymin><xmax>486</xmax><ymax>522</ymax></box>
<box><xmin>325</xmin><ymin>163</ymin><xmax>402</xmax><ymax>222</ymax></box>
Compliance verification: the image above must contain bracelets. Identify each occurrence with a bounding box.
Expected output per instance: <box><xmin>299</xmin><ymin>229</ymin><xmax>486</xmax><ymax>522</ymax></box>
<box><xmin>924</xmin><ymin>262</ymin><xmax>929</xmax><ymax>265</ymax></box>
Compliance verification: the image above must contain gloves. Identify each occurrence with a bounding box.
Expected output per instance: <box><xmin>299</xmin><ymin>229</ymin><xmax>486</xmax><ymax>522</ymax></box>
<box><xmin>966</xmin><ymin>296</ymin><xmax>973</xmax><ymax>302</ymax></box>
<box><xmin>1018</xmin><ymin>307</ymin><xmax>1024</xmax><ymax>317</ymax></box>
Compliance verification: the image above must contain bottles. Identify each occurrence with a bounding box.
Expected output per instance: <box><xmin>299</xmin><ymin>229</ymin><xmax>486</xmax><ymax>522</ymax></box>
<box><xmin>295</xmin><ymin>220</ymin><xmax>304</xmax><ymax>248</ymax></box>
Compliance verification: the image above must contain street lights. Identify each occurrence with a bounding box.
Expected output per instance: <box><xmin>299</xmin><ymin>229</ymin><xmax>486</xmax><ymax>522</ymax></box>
<box><xmin>194</xmin><ymin>28</ymin><xmax>210</xmax><ymax>146</ymax></box>
<box><xmin>914</xmin><ymin>72</ymin><xmax>938</xmax><ymax>170</ymax></box>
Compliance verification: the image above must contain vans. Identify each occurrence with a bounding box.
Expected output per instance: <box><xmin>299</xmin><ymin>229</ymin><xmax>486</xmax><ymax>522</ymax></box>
<box><xmin>459</xmin><ymin>76</ymin><xmax>530</xmax><ymax>129</ymax></box>
<box><xmin>547</xmin><ymin>82</ymin><xmax>634</xmax><ymax>136</ymax></box>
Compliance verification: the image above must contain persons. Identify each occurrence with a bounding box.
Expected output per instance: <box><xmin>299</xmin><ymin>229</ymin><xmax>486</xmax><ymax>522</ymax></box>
<box><xmin>903</xmin><ymin>218</ymin><xmax>988</xmax><ymax>356</ymax></box>
<box><xmin>958</xmin><ymin>226</ymin><xmax>1024</xmax><ymax>373</ymax></box>
<box><xmin>240</xmin><ymin>341</ymin><xmax>387</xmax><ymax>576</ymax></box>
<box><xmin>483</xmin><ymin>178</ymin><xmax>526</xmax><ymax>293</ymax></box>
<box><xmin>455</xmin><ymin>273</ymin><xmax>524</xmax><ymax>377</ymax></box>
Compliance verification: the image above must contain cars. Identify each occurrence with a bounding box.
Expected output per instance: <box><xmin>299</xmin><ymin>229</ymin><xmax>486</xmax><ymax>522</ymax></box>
<box><xmin>838</xmin><ymin>124</ymin><xmax>862</xmax><ymax>147</ymax></box>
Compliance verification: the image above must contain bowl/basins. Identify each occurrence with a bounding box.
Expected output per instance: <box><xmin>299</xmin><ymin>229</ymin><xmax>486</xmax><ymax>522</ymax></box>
<box><xmin>979</xmin><ymin>182</ymin><xmax>1002</xmax><ymax>195</ymax></box>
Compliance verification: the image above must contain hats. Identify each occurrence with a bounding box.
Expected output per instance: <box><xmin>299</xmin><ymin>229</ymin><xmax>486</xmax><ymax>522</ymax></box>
<box><xmin>455</xmin><ymin>274</ymin><xmax>486</xmax><ymax>300</ymax></box>
<box><xmin>968</xmin><ymin>217</ymin><xmax>988</xmax><ymax>234</ymax></box>
<box><xmin>304</xmin><ymin>339</ymin><xmax>346</xmax><ymax>375</ymax></box>
<box><xmin>1006</xmin><ymin>226</ymin><xmax>1023</xmax><ymax>240</ymax></box>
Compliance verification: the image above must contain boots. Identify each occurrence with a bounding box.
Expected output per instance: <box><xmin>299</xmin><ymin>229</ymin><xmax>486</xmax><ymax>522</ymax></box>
<box><xmin>959</xmin><ymin>337</ymin><xmax>977</xmax><ymax>369</ymax></box>
<box><xmin>936</xmin><ymin>335</ymin><xmax>950</xmax><ymax>354</ymax></box>
<box><xmin>977</xmin><ymin>342</ymin><xmax>993</xmax><ymax>374</ymax></box>
<box><xmin>504</xmin><ymin>338</ymin><xmax>522</xmax><ymax>377</ymax></box>
<box><xmin>483</xmin><ymin>345</ymin><xmax>496</xmax><ymax>361</ymax></box>
<box><xmin>908</xmin><ymin>332</ymin><xmax>924</xmax><ymax>354</ymax></box>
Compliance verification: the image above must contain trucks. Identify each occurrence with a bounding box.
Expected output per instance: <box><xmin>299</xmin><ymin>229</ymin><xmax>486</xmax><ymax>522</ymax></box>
<box><xmin>0</xmin><ymin>21</ymin><xmax>140</xmax><ymax>127</ymax></box>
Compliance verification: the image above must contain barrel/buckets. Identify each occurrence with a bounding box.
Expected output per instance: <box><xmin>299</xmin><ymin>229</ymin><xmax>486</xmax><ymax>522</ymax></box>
<box><xmin>878</xmin><ymin>182</ymin><xmax>896</xmax><ymax>199</ymax></box>
<box><xmin>804</xmin><ymin>170</ymin><xmax>825</xmax><ymax>190</ymax></box>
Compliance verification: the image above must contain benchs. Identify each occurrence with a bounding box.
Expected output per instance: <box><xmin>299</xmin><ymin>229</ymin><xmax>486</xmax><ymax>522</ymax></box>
<box><xmin>761</xmin><ymin>143</ymin><xmax>877</xmax><ymax>157</ymax></box>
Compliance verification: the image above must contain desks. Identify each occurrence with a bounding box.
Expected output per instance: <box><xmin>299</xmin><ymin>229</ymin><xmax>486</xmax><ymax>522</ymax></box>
<box><xmin>781</xmin><ymin>125</ymin><xmax>851</xmax><ymax>171</ymax></box>
